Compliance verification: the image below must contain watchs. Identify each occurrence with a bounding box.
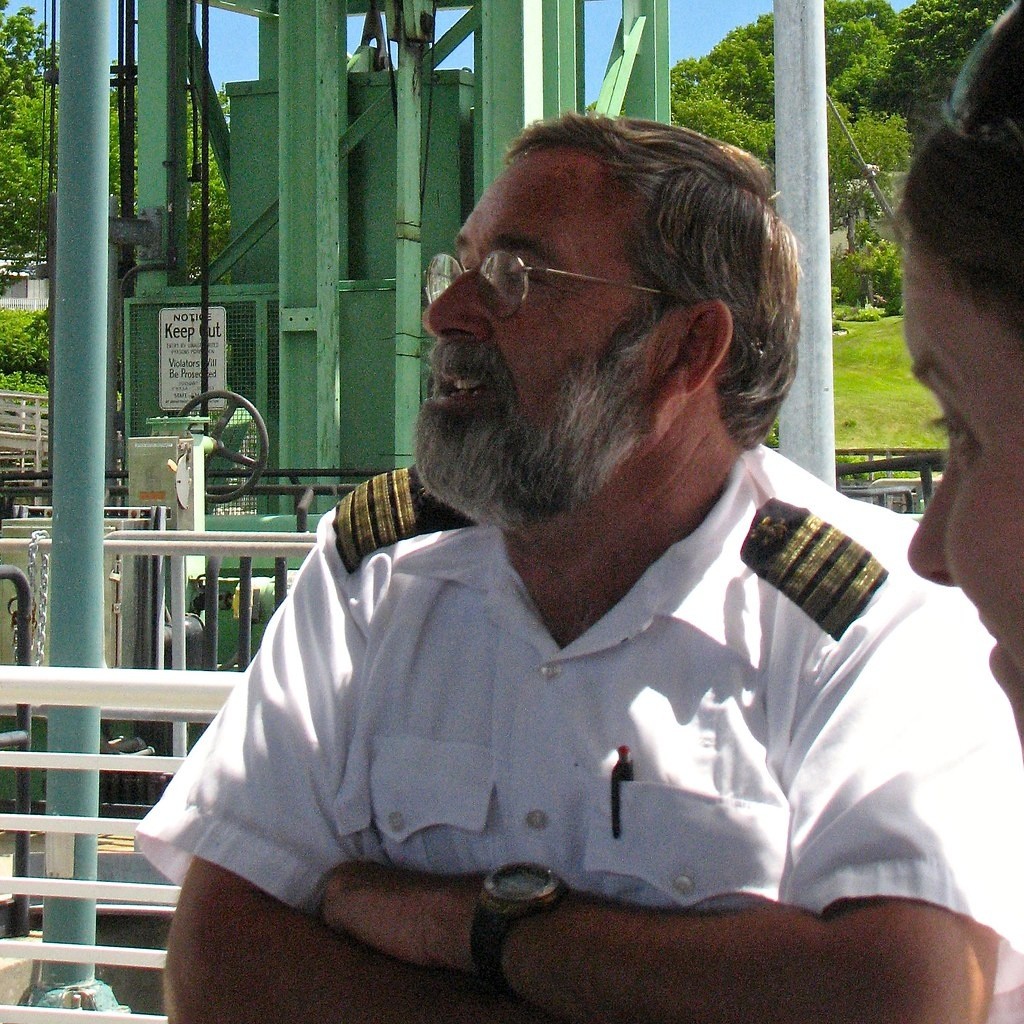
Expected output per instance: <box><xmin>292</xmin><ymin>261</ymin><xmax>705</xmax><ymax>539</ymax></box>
<box><xmin>470</xmin><ymin>861</ymin><xmax>574</xmax><ymax>1002</ymax></box>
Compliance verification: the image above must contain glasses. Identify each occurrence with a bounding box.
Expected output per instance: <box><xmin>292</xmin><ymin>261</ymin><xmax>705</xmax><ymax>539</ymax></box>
<box><xmin>425</xmin><ymin>253</ymin><xmax>681</xmax><ymax>321</ymax></box>
<box><xmin>942</xmin><ymin>0</ymin><xmax>1024</xmax><ymax>142</ymax></box>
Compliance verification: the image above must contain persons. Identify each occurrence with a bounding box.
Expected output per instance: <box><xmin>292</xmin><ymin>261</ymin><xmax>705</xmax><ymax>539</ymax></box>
<box><xmin>894</xmin><ymin>0</ymin><xmax>1024</xmax><ymax>763</ymax></box>
<box><xmin>136</xmin><ymin>111</ymin><xmax>1024</xmax><ymax>1024</ymax></box>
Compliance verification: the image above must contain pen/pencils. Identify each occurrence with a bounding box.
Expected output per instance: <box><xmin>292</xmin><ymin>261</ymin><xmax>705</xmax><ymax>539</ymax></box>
<box><xmin>608</xmin><ymin>744</ymin><xmax>636</xmax><ymax>839</ymax></box>
